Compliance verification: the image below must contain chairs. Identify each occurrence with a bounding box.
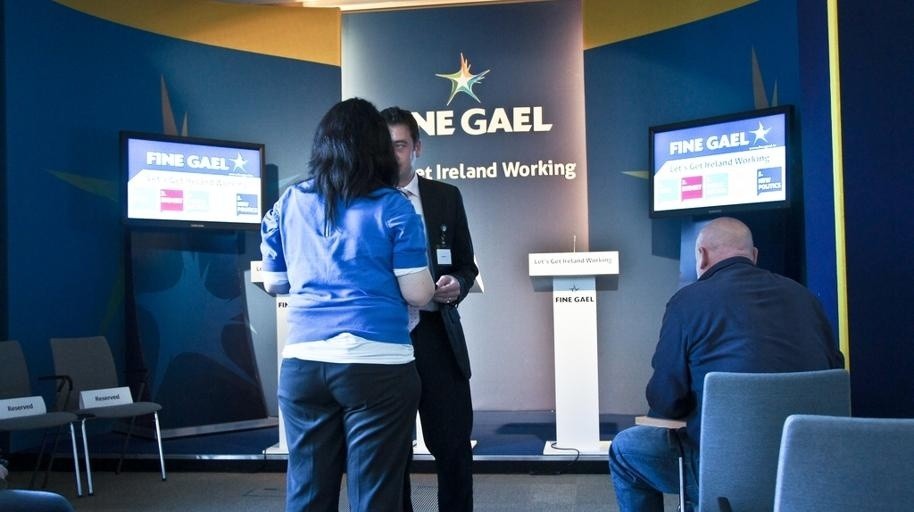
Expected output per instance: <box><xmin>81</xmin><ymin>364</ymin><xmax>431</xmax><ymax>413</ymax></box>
<box><xmin>669</xmin><ymin>369</ymin><xmax>852</xmax><ymax>512</ymax></box>
<box><xmin>0</xmin><ymin>340</ymin><xmax>83</xmax><ymax>497</ymax></box>
<box><xmin>773</xmin><ymin>414</ymin><xmax>913</xmax><ymax>512</ymax></box>
<box><xmin>0</xmin><ymin>489</ymin><xmax>75</xmax><ymax>512</ymax></box>
<box><xmin>49</xmin><ymin>335</ymin><xmax>167</xmax><ymax>496</ymax></box>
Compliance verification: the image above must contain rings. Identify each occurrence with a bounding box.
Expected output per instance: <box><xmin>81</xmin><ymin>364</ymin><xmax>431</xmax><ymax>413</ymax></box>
<box><xmin>446</xmin><ymin>298</ymin><xmax>451</xmax><ymax>304</ymax></box>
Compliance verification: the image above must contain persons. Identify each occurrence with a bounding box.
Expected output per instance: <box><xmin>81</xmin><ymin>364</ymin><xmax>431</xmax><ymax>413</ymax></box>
<box><xmin>608</xmin><ymin>214</ymin><xmax>846</xmax><ymax>512</ymax></box>
<box><xmin>261</xmin><ymin>96</ymin><xmax>436</xmax><ymax>511</ymax></box>
<box><xmin>380</xmin><ymin>105</ymin><xmax>480</xmax><ymax>511</ymax></box>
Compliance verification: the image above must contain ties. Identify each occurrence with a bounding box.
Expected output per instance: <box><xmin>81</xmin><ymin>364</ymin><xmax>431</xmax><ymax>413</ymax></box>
<box><xmin>400</xmin><ymin>189</ymin><xmax>420</xmax><ymax>334</ymax></box>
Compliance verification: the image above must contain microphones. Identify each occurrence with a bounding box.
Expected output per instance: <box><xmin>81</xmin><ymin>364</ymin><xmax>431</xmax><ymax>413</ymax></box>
<box><xmin>571</xmin><ymin>234</ymin><xmax>580</xmax><ymax>254</ymax></box>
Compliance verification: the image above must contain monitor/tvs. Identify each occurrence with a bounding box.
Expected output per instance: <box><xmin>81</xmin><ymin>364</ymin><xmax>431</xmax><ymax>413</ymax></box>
<box><xmin>650</xmin><ymin>106</ymin><xmax>795</xmax><ymax>219</ymax></box>
<box><xmin>118</xmin><ymin>128</ymin><xmax>267</xmax><ymax>231</ymax></box>
